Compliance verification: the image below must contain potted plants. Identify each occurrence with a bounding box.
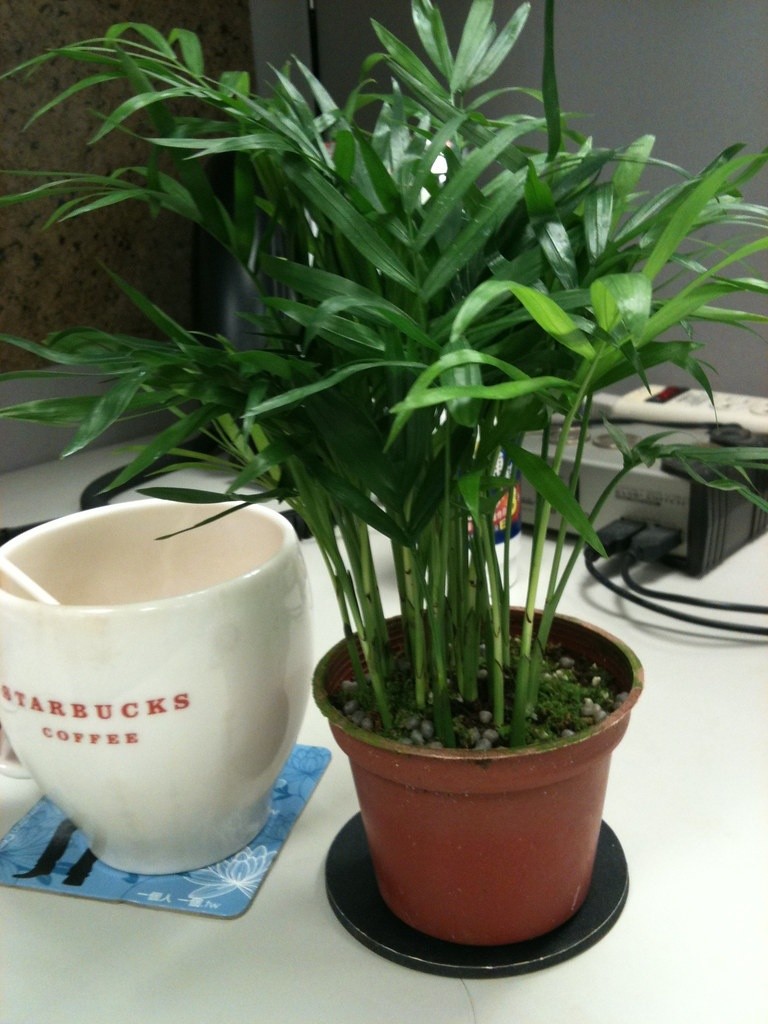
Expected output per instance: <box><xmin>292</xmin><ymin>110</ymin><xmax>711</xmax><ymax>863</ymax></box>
<box><xmin>0</xmin><ymin>0</ymin><xmax>768</xmax><ymax>950</ymax></box>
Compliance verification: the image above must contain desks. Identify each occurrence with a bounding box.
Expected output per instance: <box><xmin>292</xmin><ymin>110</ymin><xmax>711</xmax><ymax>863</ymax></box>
<box><xmin>0</xmin><ymin>434</ymin><xmax>768</xmax><ymax>1024</ymax></box>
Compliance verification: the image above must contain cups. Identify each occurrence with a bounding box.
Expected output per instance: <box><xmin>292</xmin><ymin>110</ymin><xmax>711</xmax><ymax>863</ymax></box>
<box><xmin>0</xmin><ymin>498</ymin><xmax>315</xmax><ymax>876</ymax></box>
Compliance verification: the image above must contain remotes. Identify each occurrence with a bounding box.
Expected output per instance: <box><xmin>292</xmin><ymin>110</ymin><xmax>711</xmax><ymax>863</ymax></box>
<box><xmin>612</xmin><ymin>385</ymin><xmax>768</xmax><ymax>434</ymax></box>
